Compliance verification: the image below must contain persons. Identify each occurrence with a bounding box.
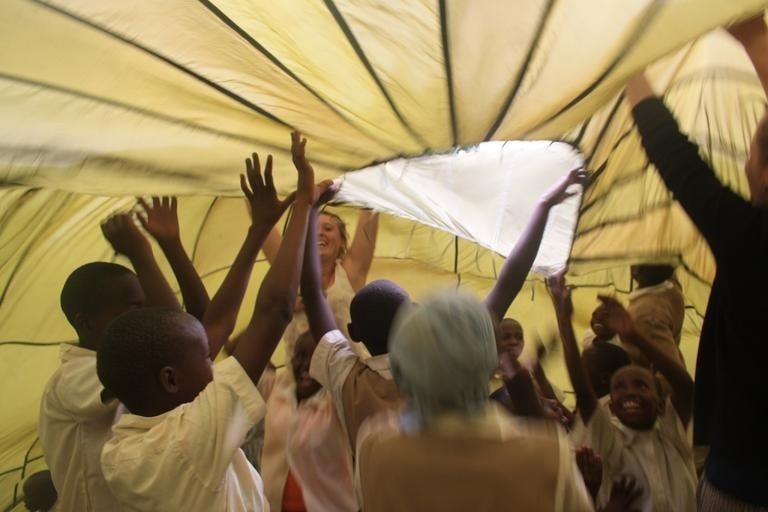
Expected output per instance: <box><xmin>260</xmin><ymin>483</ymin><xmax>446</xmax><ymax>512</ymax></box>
<box><xmin>630</xmin><ymin>14</ymin><xmax>767</xmax><ymax>512</ymax></box>
<box><xmin>22</xmin><ymin>131</ymin><xmax>699</xmax><ymax>510</ymax></box>
